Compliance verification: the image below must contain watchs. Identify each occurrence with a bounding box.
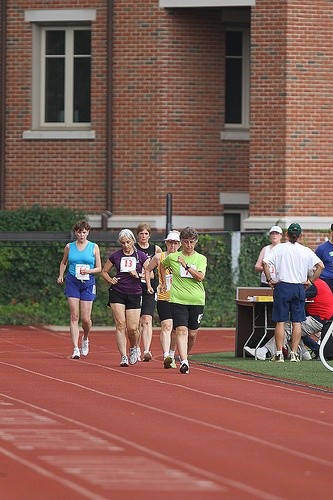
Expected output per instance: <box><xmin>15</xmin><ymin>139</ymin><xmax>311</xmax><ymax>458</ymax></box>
<box><xmin>185</xmin><ymin>263</ymin><xmax>191</xmax><ymax>270</ymax></box>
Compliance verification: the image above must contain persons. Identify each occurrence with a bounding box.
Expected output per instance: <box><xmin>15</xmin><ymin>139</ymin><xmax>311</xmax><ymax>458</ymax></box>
<box><xmin>244</xmin><ymin>266</ymin><xmax>333</xmax><ymax>361</ymax></box>
<box><xmin>101</xmin><ymin>228</ymin><xmax>154</xmax><ymax>365</ymax></box>
<box><xmin>160</xmin><ymin>226</ymin><xmax>207</xmax><ymax>373</ymax></box>
<box><xmin>262</xmin><ymin>223</ymin><xmax>325</xmax><ymax>362</ymax></box>
<box><xmin>133</xmin><ymin>224</ymin><xmax>163</xmax><ymax>362</ymax></box>
<box><xmin>315</xmin><ymin>224</ymin><xmax>333</xmax><ymax>293</ymax></box>
<box><xmin>255</xmin><ymin>226</ymin><xmax>283</xmax><ymax>287</ymax></box>
<box><xmin>301</xmin><ymin>334</ymin><xmax>321</xmax><ymax>360</ymax></box>
<box><xmin>144</xmin><ymin>229</ymin><xmax>181</xmax><ymax>369</ymax></box>
<box><xmin>56</xmin><ymin>220</ymin><xmax>102</xmax><ymax>359</ymax></box>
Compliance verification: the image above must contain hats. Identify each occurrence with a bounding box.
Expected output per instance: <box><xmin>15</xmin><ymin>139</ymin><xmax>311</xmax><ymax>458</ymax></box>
<box><xmin>288</xmin><ymin>223</ymin><xmax>301</xmax><ymax>237</ymax></box>
<box><xmin>163</xmin><ymin>231</ymin><xmax>180</xmax><ymax>242</ymax></box>
<box><xmin>269</xmin><ymin>226</ymin><xmax>282</xmax><ymax>234</ymax></box>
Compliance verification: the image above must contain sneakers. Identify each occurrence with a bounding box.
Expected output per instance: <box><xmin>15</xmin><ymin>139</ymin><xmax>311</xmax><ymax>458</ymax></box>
<box><xmin>289</xmin><ymin>353</ymin><xmax>300</xmax><ymax>362</ymax></box>
<box><xmin>244</xmin><ymin>346</ymin><xmax>266</xmax><ymax>360</ymax></box>
<box><xmin>120</xmin><ymin>356</ymin><xmax>128</xmax><ymax>367</ymax></box>
<box><xmin>143</xmin><ymin>350</ymin><xmax>151</xmax><ymax>362</ymax></box>
<box><xmin>269</xmin><ymin>353</ymin><xmax>284</xmax><ymax>362</ymax></box>
<box><xmin>72</xmin><ymin>349</ymin><xmax>80</xmax><ymax>359</ymax></box>
<box><xmin>170</xmin><ymin>357</ymin><xmax>177</xmax><ymax>369</ymax></box>
<box><xmin>303</xmin><ymin>351</ymin><xmax>312</xmax><ymax>360</ymax></box>
<box><xmin>81</xmin><ymin>335</ymin><xmax>89</xmax><ymax>356</ymax></box>
<box><xmin>179</xmin><ymin>362</ymin><xmax>189</xmax><ymax>374</ymax></box>
<box><xmin>136</xmin><ymin>346</ymin><xmax>141</xmax><ymax>361</ymax></box>
<box><xmin>129</xmin><ymin>348</ymin><xmax>137</xmax><ymax>364</ymax></box>
<box><xmin>164</xmin><ymin>356</ymin><xmax>172</xmax><ymax>368</ymax></box>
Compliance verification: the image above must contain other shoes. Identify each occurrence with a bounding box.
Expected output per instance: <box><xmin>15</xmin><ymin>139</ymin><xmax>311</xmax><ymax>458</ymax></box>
<box><xmin>311</xmin><ymin>349</ymin><xmax>320</xmax><ymax>359</ymax></box>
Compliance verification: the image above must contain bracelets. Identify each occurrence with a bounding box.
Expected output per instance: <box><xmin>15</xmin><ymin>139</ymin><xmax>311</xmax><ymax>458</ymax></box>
<box><xmin>266</xmin><ymin>277</ymin><xmax>272</xmax><ymax>282</ymax></box>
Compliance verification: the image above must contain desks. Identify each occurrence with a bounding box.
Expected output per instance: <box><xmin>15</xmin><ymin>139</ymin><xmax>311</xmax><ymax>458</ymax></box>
<box><xmin>236</xmin><ymin>299</ymin><xmax>315</xmax><ymax>361</ymax></box>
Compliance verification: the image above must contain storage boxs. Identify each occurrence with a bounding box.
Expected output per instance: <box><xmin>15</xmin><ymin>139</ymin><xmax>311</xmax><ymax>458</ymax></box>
<box><xmin>253</xmin><ymin>295</ymin><xmax>274</xmax><ymax>302</ymax></box>
